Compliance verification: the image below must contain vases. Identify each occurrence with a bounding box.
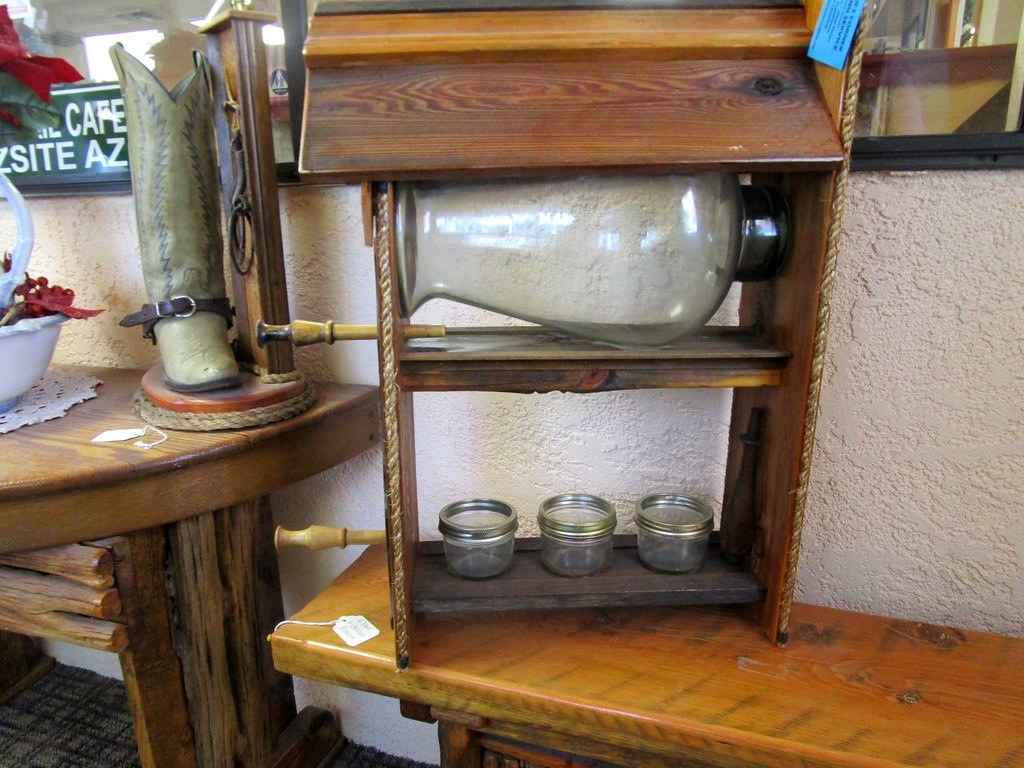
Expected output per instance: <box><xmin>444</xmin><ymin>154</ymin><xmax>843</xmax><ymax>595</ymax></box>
<box><xmin>0</xmin><ymin>172</ymin><xmax>74</xmax><ymax>413</ymax></box>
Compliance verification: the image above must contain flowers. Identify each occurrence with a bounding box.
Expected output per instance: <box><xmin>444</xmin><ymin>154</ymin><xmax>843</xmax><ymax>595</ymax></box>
<box><xmin>0</xmin><ymin>4</ymin><xmax>85</xmax><ymax>151</ymax></box>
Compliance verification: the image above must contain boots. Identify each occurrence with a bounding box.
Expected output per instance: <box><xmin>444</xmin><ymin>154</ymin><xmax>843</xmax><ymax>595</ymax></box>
<box><xmin>108</xmin><ymin>43</ymin><xmax>243</xmax><ymax>392</ymax></box>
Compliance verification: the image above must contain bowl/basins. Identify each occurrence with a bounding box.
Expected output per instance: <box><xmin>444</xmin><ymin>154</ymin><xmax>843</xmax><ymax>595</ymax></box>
<box><xmin>0</xmin><ymin>313</ymin><xmax>70</xmax><ymax>415</ymax></box>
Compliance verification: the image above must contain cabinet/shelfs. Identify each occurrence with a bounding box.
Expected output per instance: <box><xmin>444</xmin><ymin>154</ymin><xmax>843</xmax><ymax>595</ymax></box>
<box><xmin>290</xmin><ymin>0</ymin><xmax>848</xmax><ymax>682</ymax></box>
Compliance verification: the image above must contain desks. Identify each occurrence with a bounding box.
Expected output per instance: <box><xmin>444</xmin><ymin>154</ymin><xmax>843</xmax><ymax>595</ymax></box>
<box><xmin>1</xmin><ymin>364</ymin><xmax>389</xmax><ymax>768</ymax></box>
<box><xmin>272</xmin><ymin>542</ymin><xmax>1024</xmax><ymax>768</ymax></box>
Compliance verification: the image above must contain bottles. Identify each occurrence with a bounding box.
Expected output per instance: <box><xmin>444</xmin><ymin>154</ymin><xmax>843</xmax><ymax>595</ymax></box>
<box><xmin>634</xmin><ymin>493</ymin><xmax>714</xmax><ymax>573</ymax></box>
<box><xmin>538</xmin><ymin>494</ymin><xmax>616</xmax><ymax>577</ymax></box>
<box><xmin>393</xmin><ymin>170</ymin><xmax>790</xmax><ymax>349</ymax></box>
<box><xmin>439</xmin><ymin>499</ymin><xmax>518</xmax><ymax>578</ymax></box>
